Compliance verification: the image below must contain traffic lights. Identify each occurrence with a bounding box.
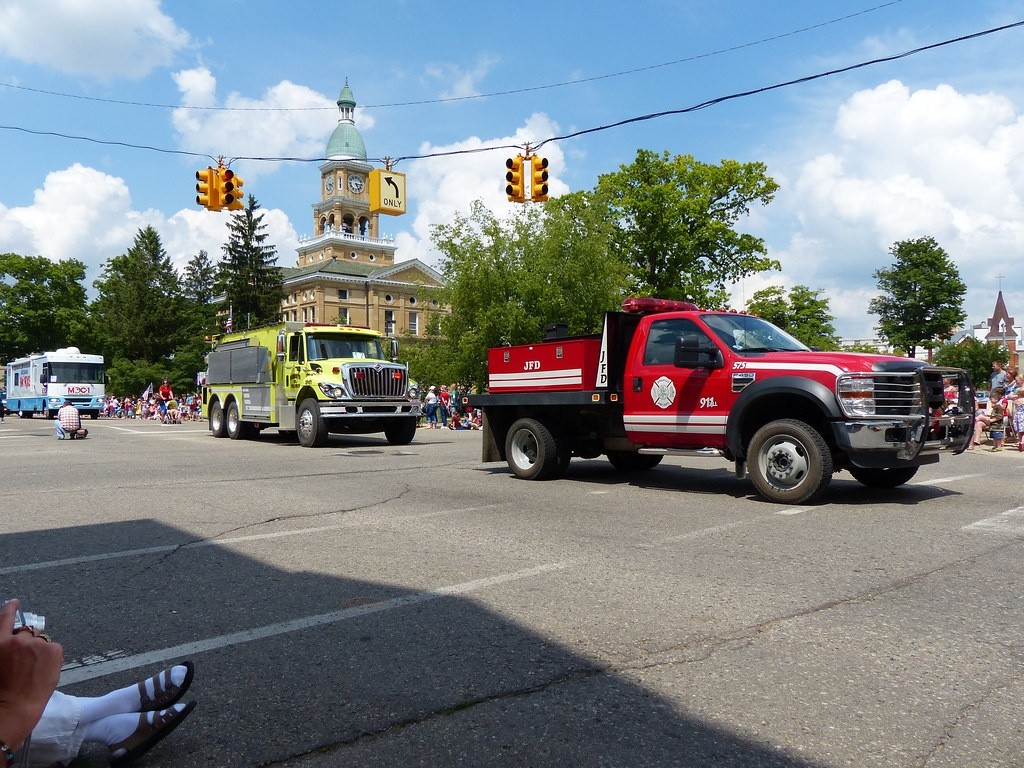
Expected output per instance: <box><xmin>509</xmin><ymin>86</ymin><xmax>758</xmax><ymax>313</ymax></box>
<box><xmin>505</xmin><ymin>158</ymin><xmax>522</xmax><ymax>200</ymax></box>
<box><xmin>234</xmin><ymin>176</ymin><xmax>244</xmax><ymax>211</ymax></box>
<box><xmin>531</xmin><ymin>155</ymin><xmax>549</xmax><ymax>198</ymax></box>
<box><xmin>218</xmin><ymin>171</ymin><xmax>236</xmax><ymax>208</ymax></box>
<box><xmin>196</xmin><ymin>169</ymin><xmax>212</xmax><ymax>208</ymax></box>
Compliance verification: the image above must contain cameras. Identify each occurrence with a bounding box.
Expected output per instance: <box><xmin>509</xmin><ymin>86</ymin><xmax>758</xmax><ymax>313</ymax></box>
<box><xmin>4</xmin><ymin>601</ymin><xmax>45</xmax><ymax>633</ymax></box>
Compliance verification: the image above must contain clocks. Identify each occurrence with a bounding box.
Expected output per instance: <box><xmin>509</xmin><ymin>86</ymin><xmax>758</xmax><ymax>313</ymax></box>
<box><xmin>325</xmin><ymin>175</ymin><xmax>335</xmax><ymax>193</ymax></box>
<box><xmin>347</xmin><ymin>174</ymin><xmax>365</xmax><ymax>193</ymax></box>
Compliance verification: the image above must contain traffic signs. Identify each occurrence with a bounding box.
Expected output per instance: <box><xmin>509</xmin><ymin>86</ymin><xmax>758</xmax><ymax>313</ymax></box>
<box><xmin>369</xmin><ymin>169</ymin><xmax>406</xmax><ymax>216</ymax></box>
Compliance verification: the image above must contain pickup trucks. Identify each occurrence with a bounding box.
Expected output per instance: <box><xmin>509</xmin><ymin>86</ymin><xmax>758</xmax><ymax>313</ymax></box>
<box><xmin>454</xmin><ymin>298</ymin><xmax>976</xmax><ymax>504</ymax></box>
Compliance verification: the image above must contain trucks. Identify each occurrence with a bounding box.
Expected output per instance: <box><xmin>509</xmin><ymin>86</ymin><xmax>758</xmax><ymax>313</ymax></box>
<box><xmin>6</xmin><ymin>346</ymin><xmax>112</xmax><ymax>420</ymax></box>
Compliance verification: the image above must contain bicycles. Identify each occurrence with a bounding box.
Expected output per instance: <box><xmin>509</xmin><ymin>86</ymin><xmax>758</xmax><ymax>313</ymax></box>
<box><xmin>105</xmin><ymin>408</ymin><xmax>124</xmax><ymax>419</ymax></box>
<box><xmin>183</xmin><ymin>411</ymin><xmax>203</xmax><ymax>421</ymax></box>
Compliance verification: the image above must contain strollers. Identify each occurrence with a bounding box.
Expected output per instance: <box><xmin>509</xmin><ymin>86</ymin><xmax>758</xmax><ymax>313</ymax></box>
<box><xmin>164</xmin><ymin>399</ymin><xmax>182</xmax><ymax>425</ymax></box>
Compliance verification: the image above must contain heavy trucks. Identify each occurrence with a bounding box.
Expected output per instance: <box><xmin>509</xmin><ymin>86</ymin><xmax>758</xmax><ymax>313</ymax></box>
<box><xmin>198</xmin><ymin>320</ymin><xmax>422</xmax><ymax>447</ymax></box>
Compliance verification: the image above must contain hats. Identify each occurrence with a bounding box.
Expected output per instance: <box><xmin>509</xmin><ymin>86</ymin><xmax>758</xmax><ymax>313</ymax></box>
<box><xmin>429</xmin><ymin>385</ymin><xmax>436</xmax><ymax>389</ymax></box>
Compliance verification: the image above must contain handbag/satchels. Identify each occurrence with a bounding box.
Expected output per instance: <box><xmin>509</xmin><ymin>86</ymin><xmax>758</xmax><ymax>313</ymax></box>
<box><xmin>76</xmin><ymin>428</ymin><xmax>88</xmax><ymax>439</ymax></box>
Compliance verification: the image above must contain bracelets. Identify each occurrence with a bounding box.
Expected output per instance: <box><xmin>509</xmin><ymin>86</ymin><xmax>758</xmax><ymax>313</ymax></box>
<box><xmin>0</xmin><ymin>740</ymin><xmax>16</xmax><ymax>766</ymax></box>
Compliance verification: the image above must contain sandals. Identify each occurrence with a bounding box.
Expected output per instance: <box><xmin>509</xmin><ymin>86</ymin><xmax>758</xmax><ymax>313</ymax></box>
<box><xmin>107</xmin><ymin>700</ymin><xmax>197</xmax><ymax>765</ymax></box>
<box><xmin>138</xmin><ymin>660</ymin><xmax>194</xmax><ymax>711</ymax></box>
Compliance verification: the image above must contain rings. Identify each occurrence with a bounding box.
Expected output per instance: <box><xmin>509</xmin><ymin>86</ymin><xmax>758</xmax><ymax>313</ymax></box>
<box><xmin>14</xmin><ymin>624</ymin><xmax>35</xmax><ymax>637</ymax></box>
<box><xmin>37</xmin><ymin>633</ymin><xmax>52</xmax><ymax>642</ymax></box>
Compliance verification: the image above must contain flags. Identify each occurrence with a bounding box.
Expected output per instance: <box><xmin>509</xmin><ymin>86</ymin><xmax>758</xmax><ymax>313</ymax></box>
<box><xmin>142</xmin><ymin>384</ymin><xmax>153</xmax><ymax>400</ymax></box>
<box><xmin>226</xmin><ymin>318</ymin><xmax>232</xmax><ymax>333</ymax></box>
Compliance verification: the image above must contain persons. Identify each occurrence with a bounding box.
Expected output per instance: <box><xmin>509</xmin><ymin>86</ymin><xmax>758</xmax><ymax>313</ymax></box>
<box><xmin>409</xmin><ymin>384</ymin><xmax>484</xmax><ymax>430</ymax></box>
<box><xmin>99</xmin><ymin>379</ymin><xmax>202</xmax><ymax>424</ymax></box>
<box><xmin>929</xmin><ymin>359</ymin><xmax>1024</xmax><ymax>452</ymax></box>
<box><xmin>0</xmin><ymin>399</ymin><xmax>5</xmax><ymax>423</ymax></box>
<box><xmin>55</xmin><ymin>400</ymin><xmax>82</xmax><ymax>440</ymax></box>
<box><xmin>0</xmin><ymin>598</ymin><xmax>198</xmax><ymax>768</ymax></box>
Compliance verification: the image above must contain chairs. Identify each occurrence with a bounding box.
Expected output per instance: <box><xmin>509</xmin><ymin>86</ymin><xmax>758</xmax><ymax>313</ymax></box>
<box><xmin>984</xmin><ymin>409</ymin><xmax>1017</xmax><ymax>442</ymax></box>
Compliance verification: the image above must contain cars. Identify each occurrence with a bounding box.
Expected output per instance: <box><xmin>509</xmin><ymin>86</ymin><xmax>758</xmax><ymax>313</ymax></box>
<box><xmin>976</xmin><ymin>391</ymin><xmax>991</xmax><ymax>401</ymax></box>
<box><xmin>2</xmin><ymin>393</ymin><xmax>7</xmax><ymax>413</ymax></box>
